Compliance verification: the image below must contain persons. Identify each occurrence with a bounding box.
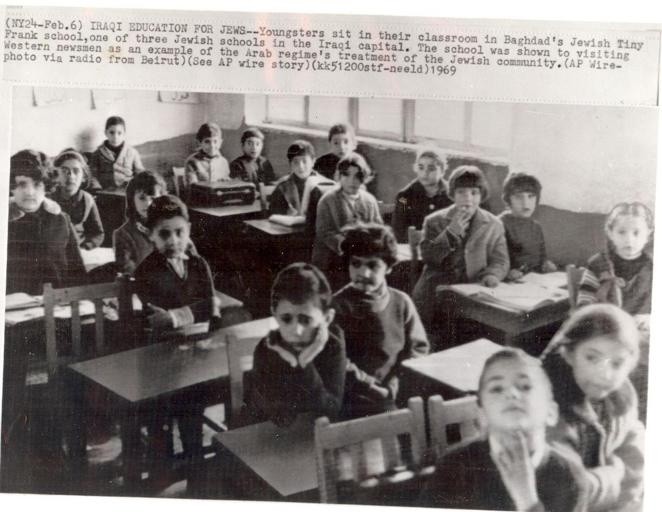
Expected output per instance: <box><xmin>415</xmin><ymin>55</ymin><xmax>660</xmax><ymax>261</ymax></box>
<box><xmin>576</xmin><ymin>202</ymin><xmax>655</xmax><ymax>315</ymax></box>
<box><xmin>417</xmin><ymin>347</ymin><xmax>590</xmax><ymax>512</ymax></box>
<box><xmin>534</xmin><ymin>303</ymin><xmax>645</xmax><ymax>512</ymax></box>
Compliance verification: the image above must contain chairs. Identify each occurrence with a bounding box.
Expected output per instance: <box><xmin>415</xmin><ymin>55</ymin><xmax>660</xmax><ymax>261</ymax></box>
<box><xmin>1</xmin><ymin>156</ymin><xmax>654</xmax><ymax>508</ymax></box>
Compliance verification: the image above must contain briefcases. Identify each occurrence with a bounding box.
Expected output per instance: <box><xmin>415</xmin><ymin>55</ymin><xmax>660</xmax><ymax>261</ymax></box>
<box><xmin>190</xmin><ymin>177</ymin><xmax>256</xmax><ymax>208</ymax></box>
